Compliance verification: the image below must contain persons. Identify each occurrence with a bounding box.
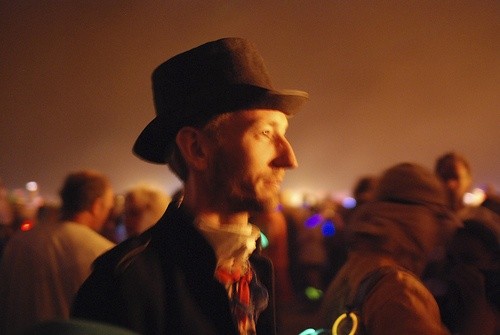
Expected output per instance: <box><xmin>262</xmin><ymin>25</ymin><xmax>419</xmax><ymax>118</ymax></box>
<box><xmin>69</xmin><ymin>37</ymin><xmax>310</xmax><ymax>335</ymax></box>
<box><xmin>0</xmin><ymin>154</ymin><xmax>500</xmax><ymax>335</ymax></box>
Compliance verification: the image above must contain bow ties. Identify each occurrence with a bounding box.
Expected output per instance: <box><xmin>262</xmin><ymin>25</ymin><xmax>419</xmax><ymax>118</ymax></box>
<box><xmin>217</xmin><ymin>260</ymin><xmax>256</xmax><ymax>335</ymax></box>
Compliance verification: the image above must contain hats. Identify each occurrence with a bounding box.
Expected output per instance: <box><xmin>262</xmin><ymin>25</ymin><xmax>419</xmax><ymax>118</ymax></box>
<box><xmin>367</xmin><ymin>163</ymin><xmax>463</xmax><ymax>227</ymax></box>
<box><xmin>132</xmin><ymin>37</ymin><xmax>310</xmax><ymax>165</ymax></box>
